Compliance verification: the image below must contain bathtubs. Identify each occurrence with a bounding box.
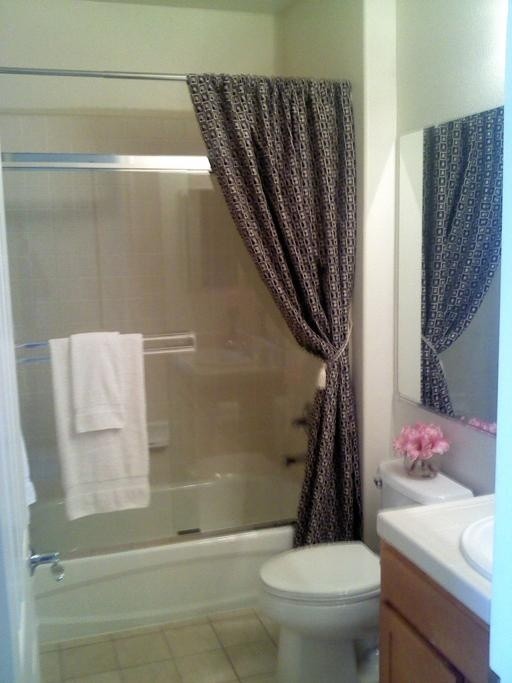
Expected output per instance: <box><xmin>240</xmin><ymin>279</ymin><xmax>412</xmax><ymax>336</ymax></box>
<box><xmin>32</xmin><ymin>463</ymin><xmax>323</xmax><ymax>646</ymax></box>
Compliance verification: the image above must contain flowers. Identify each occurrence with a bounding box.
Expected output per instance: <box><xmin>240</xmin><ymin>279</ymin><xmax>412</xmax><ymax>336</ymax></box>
<box><xmin>462</xmin><ymin>416</ymin><xmax>496</xmax><ymax>435</ymax></box>
<box><xmin>394</xmin><ymin>422</ymin><xmax>450</xmax><ymax>461</ymax></box>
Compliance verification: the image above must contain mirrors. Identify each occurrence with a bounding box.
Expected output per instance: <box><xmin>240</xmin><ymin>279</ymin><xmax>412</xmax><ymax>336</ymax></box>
<box><xmin>398</xmin><ymin>106</ymin><xmax>504</xmax><ymax>436</ymax></box>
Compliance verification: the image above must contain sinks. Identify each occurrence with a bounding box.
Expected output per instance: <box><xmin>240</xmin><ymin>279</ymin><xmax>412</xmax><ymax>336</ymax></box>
<box><xmin>461</xmin><ymin>514</ymin><xmax>493</xmax><ymax>575</ymax></box>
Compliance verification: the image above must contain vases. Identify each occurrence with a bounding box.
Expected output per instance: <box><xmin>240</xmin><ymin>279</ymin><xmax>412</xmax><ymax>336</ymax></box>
<box><xmin>404</xmin><ymin>459</ymin><xmax>438</xmax><ymax>479</ymax></box>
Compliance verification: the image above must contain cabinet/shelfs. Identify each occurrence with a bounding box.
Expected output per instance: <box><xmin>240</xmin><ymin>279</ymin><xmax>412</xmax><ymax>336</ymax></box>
<box><xmin>379</xmin><ymin>541</ymin><xmax>500</xmax><ymax>683</ymax></box>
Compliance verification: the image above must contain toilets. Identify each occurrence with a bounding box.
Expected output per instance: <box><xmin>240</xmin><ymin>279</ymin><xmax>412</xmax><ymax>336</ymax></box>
<box><xmin>259</xmin><ymin>458</ymin><xmax>473</xmax><ymax>683</ymax></box>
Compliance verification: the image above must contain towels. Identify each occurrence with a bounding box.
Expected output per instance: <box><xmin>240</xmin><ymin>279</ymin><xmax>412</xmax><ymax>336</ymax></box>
<box><xmin>48</xmin><ymin>333</ymin><xmax>151</xmax><ymax>521</ymax></box>
<box><xmin>70</xmin><ymin>332</ymin><xmax>126</xmax><ymax>435</ymax></box>
